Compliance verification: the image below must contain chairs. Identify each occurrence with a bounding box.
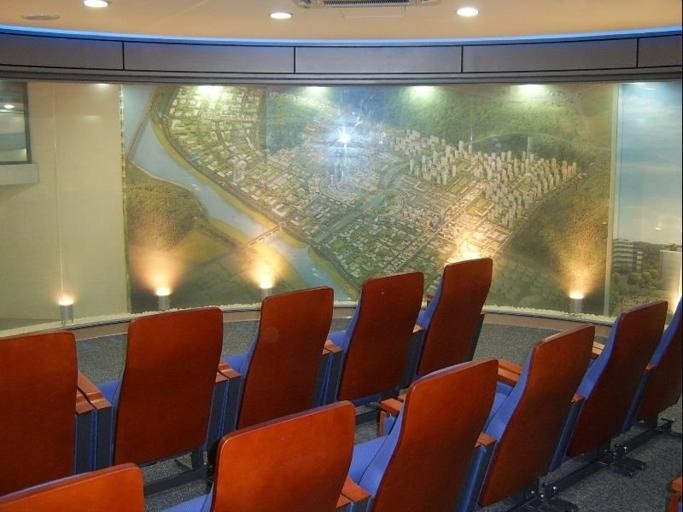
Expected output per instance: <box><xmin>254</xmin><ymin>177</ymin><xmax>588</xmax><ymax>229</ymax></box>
<box><xmin>406</xmin><ymin>257</ymin><xmax>495</xmax><ymax>387</ymax></box>
<box><xmin>620</xmin><ymin>296</ymin><xmax>682</xmax><ymax>454</ymax></box>
<box><xmin>77</xmin><ymin>303</ymin><xmax>229</xmax><ymax>483</ymax></box>
<box><xmin>219</xmin><ymin>283</ymin><xmax>335</xmax><ymax>430</ymax></box>
<box><xmin>342</xmin><ymin>358</ymin><xmax>501</xmax><ymax>512</ymax></box>
<box><xmin>399</xmin><ymin>322</ymin><xmax>594</xmax><ymax>512</ymax></box>
<box><xmin>325</xmin><ymin>273</ymin><xmax>425</xmax><ymax>410</ymax></box>
<box><xmin>495</xmin><ymin>294</ymin><xmax>670</xmax><ymax>479</ymax></box>
<box><xmin>160</xmin><ymin>397</ymin><xmax>357</xmax><ymax>511</ymax></box>
<box><xmin>2</xmin><ymin>330</ymin><xmax>96</xmax><ymax>497</ymax></box>
<box><xmin>2</xmin><ymin>460</ymin><xmax>144</xmax><ymax>512</ymax></box>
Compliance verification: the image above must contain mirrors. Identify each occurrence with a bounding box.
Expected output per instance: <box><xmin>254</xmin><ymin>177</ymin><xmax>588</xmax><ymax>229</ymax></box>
<box><xmin>0</xmin><ymin>80</ymin><xmax>32</xmax><ymax>165</ymax></box>
